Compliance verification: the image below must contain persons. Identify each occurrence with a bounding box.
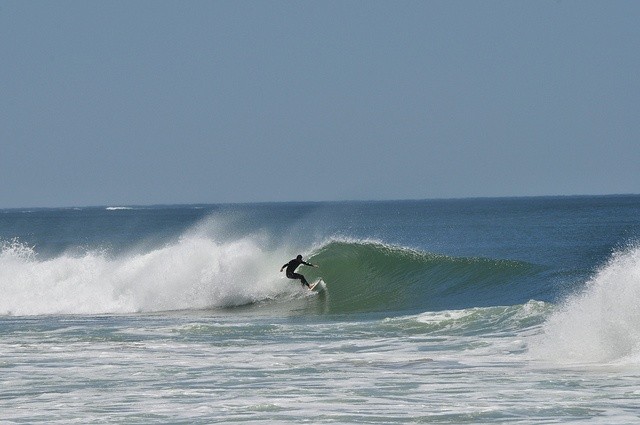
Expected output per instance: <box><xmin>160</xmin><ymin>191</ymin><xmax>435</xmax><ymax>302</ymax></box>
<box><xmin>279</xmin><ymin>254</ymin><xmax>320</xmax><ymax>289</ymax></box>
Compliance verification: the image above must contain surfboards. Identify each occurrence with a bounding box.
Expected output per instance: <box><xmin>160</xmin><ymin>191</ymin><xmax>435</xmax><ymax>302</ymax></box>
<box><xmin>308</xmin><ymin>279</ymin><xmax>321</xmax><ymax>291</ymax></box>
